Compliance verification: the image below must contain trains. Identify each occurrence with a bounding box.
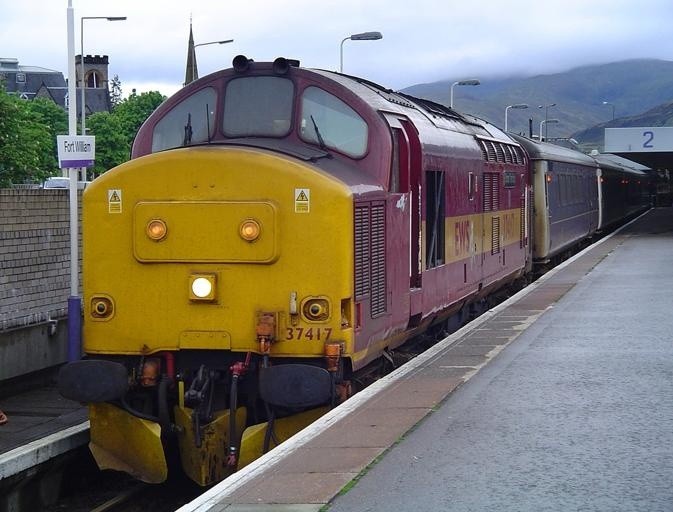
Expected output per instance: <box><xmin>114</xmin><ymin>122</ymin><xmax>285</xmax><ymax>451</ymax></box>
<box><xmin>52</xmin><ymin>55</ymin><xmax>658</xmax><ymax>488</ymax></box>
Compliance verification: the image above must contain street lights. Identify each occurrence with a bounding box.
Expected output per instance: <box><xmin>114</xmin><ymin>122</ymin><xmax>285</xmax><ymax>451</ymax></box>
<box><xmin>539</xmin><ymin>119</ymin><xmax>558</xmax><ymax>143</ymax></box>
<box><xmin>337</xmin><ymin>32</ymin><xmax>382</xmax><ymax>75</ymax></box>
<box><xmin>603</xmin><ymin>100</ymin><xmax>614</xmax><ymax>121</ymax></box>
<box><xmin>503</xmin><ymin>103</ymin><xmax>530</xmax><ymax>130</ymax></box>
<box><xmin>79</xmin><ymin>14</ymin><xmax>128</xmax><ymax>181</ymax></box>
<box><xmin>191</xmin><ymin>39</ymin><xmax>235</xmax><ymax>80</ymax></box>
<box><xmin>538</xmin><ymin>103</ymin><xmax>556</xmax><ymax>141</ymax></box>
<box><xmin>448</xmin><ymin>78</ymin><xmax>480</xmax><ymax>110</ymax></box>
